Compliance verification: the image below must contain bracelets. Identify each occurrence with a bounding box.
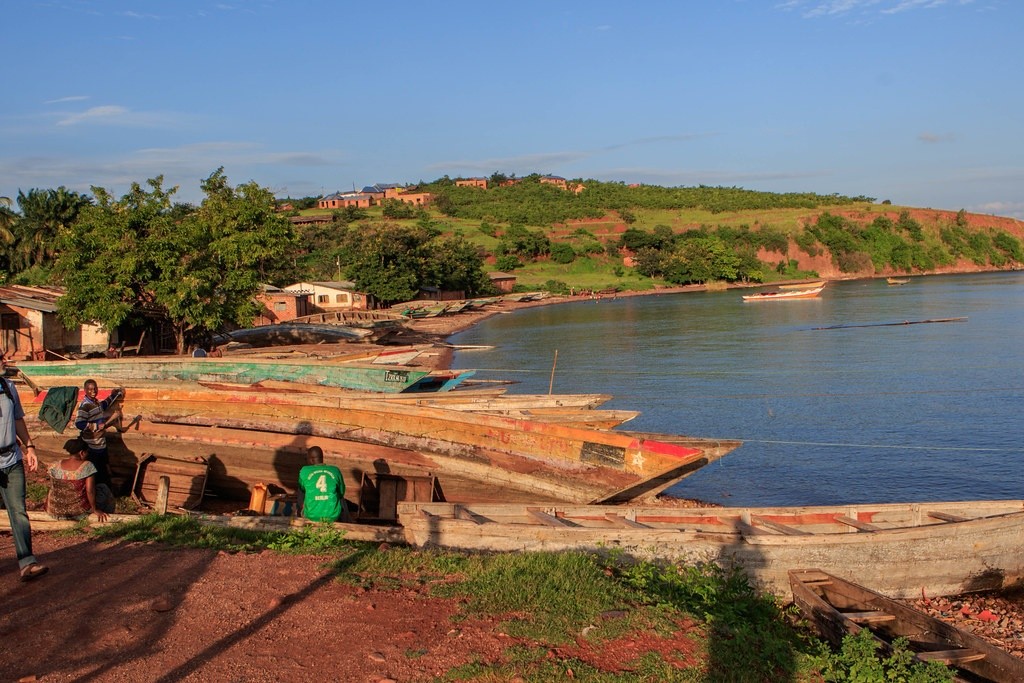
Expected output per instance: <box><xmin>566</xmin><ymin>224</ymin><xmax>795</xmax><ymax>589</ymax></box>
<box><xmin>26</xmin><ymin>445</ymin><xmax>35</xmax><ymax>448</ymax></box>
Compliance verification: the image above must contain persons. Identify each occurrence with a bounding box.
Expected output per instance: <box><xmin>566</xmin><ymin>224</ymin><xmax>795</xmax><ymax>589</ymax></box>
<box><xmin>106</xmin><ymin>344</ymin><xmax>119</xmax><ymax>358</ymax></box>
<box><xmin>0</xmin><ymin>349</ymin><xmax>49</xmax><ymax>582</ymax></box>
<box><xmin>192</xmin><ymin>344</ymin><xmax>207</xmax><ymax>357</ymax></box>
<box><xmin>208</xmin><ymin>345</ymin><xmax>222</xmax><ymax>358</ymax></box>
<box><xmin>298</xmin><ymin>446</ymin><xmax>353</xmax><ymax>524</ymax></box>
<box><xmin>75</xmin><ymin>379</ymin><xmax>120</xmax><ymax>490</ymax></box>
<box><xmin>43</xmin><ymin>438</ymin><xmax>110</xmax><ymax>522</ymax></box>
<box><xmin>569</xmin><ymin>284</ymin><xmax>601</xmax><ymax>297</ymax></box>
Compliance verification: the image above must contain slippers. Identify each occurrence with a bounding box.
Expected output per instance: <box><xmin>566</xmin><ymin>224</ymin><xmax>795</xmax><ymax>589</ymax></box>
<box><xmin>20</xmin><ymin>564</ymin><xmax>48</xmax><ymax>582</ymax></box>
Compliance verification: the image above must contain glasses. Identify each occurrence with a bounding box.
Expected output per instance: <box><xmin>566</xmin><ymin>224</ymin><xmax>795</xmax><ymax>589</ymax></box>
<box><xmin>0</xmin><ymin>354</ymin><xmax>4</xmax><ymax>361</ymax></box>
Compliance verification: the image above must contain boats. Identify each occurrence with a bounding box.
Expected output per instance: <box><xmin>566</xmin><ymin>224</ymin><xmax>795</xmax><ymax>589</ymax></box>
<box><xmin>777</xmin><ymin>280</ymin><xmax>826</xmax><ymax>289</ymax></box>
<box><xmin>392</xmin><ymin>495</ymin><xmax>1023</xmax><ymax>597</ymax></box>
<box><xmin>742</xmin><ymin>286</ymin><xmax>825</xmax><ymax>301</ymax></box>
<box><xmin>786</xmin><ymin>568</ymin><xmax>1023</xmax><ymax>681</ymax></box>
<box><xmin>0</xmin><ymin>295</ymin><xmax>746</xmax><ymax>537</ymax></box>
<box><xmin>886</xmin><ymin>276</ymin><xmax>910</xmax><ymax>286</ymax></box>
